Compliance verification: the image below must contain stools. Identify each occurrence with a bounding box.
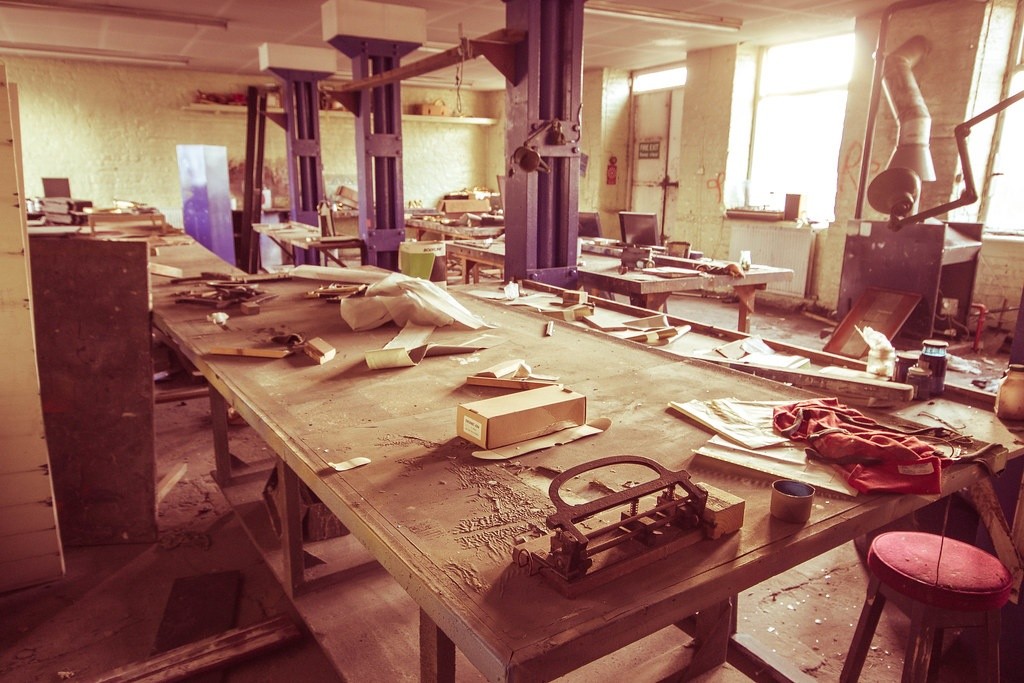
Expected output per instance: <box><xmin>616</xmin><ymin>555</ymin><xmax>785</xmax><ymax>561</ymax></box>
<box><xmin>840</xmin><ymin>531</ymin><xmax>1013</xmax><ymax>683</ymax></box>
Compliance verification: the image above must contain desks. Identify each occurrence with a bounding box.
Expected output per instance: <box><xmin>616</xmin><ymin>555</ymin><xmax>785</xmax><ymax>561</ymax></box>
<box><xmin>25</xmin><ymin>225</ymin><xmax>81</xmax><ymax>236</ymax></box>
<box><xmin>87</xmin><ymin>208</ymin><xmax>167</xmax><ymax>237</ymax></box>
<box><xmin>151</xmin><ymin>266</ymin><xmax>1008</xmax><ymax>683</ymax></box>
<box><xmin>403</xmin><ymin>216</ymin><xmax>505</xmax><ymax>241</ymax></box>
<box><xmin>445</xmin><ymin>236</ymin><xmax>795</xmax><ymax>334</ymax></box>
<box><xmin>80</xmin><ymin>219</ymin><xmax>250</xmax><ymax>289</ymax></box>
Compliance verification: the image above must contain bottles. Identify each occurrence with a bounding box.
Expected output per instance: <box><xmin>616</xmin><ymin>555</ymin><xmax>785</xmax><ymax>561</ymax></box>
<box><xmin>993</xmin><ymin>364</ymin><xmax>1024</xmax><ymax>421</ymax></box>
<box><xmin>261</xmin><ymin>187</ymin><xmax>271</xmax><ymax>210</ymax></box>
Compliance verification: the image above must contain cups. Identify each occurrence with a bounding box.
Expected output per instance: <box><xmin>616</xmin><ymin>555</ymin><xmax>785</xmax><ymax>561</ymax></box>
<box><xmin>770</xmin><ymin>480</ymin><xmax>815</xmax><ymax>524</ymax></box>
<box><xmin>740</xmin><ymin>251</ymin><xmax>751</xmax><ymax>271</ymax></box>
<box><xmin>867</xmin><ymin>348</ymin><xmax>896</xmax><ymax>376</ymax></box>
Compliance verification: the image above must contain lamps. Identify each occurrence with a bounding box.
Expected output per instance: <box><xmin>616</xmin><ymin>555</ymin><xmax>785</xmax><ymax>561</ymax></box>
<box><xmin>866</xmin><ymin>91</ymin><xmax>1024</xmax><ymax>229</ymax></box>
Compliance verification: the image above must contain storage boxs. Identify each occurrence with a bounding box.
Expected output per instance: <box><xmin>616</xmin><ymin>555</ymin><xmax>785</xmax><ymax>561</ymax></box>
<box><xmin>455</xmin><ymin>382</ymin><xmax>586</xmax><ymax>449</ymax></box>
<box><xmin>438</xmin><ymin>199</ymin><xmax>491</xmax><ymax>219</ymax></box>
